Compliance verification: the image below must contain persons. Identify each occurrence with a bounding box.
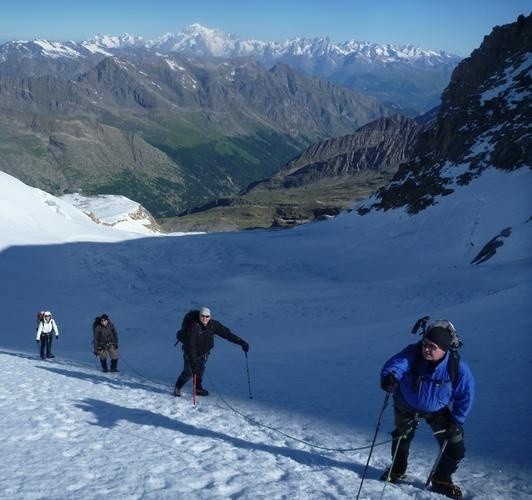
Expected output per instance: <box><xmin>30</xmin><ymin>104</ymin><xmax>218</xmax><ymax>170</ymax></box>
<box><xmin>35</xmin><ymin>311</ymin><xmax>59</xmax><ymax>360</ymax></box>
<box><xmin>173</xmin><ymin>306</ymin><xmax>249</xmax><ymax>397</ymax></box>
<box><xmin>93</xmin><ymin>314</ymin><xmax>119</xmax><ymax>372</ymax></box>
<box><xmin>379</xmin><ymin>319</ymin><xmax>475</xmax><ymax>500</ymax></box>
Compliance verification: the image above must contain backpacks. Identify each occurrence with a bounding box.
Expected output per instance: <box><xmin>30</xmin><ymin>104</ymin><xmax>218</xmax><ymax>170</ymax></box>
<box><xmin>176</xmin><ymin>310</ymin><xmax>201</xmax><ymax>344</ymax></box>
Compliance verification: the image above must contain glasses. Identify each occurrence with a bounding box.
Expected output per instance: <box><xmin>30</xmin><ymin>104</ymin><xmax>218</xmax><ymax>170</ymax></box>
<box><xmin>421</xmin><ymin>339</ymin><xmax>436</xmax><ymax>350</ymax></box>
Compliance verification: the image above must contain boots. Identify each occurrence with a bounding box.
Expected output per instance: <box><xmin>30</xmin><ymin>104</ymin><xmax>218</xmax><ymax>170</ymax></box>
<box><xmin>100</xmin><ymin>358</ymin><xmax>107</xmax><ymax>371</ymax></box>
<box><xmin>111</xmin><ymin>358</ymin><xmax>117</xmax><ymax>371</ymax></box>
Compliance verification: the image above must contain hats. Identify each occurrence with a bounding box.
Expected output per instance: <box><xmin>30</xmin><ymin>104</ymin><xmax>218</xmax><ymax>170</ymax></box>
<box><xmin>101</xmin><ymin>314</ymin><xmax>108</xmax><ymax>319</ymax></box>
<box><xmin>200</xmin><ymin>307</ymin><xmax>210</xmax><ymax>316</ymax></box>
<box><xmin>425</xmin><ymin>327</ymin><xmax>452</xmax><ymax>353</ymax></box>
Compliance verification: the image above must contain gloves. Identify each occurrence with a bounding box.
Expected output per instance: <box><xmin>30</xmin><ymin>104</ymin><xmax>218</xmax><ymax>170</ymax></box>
<box><xmin>446</xmin><ymin>425</ymin><xmax>462</xmax><ymax>443</ymax></box>
<box><xmin>241</xmin><ymin>342</ymin><xmax>249</xmax><ymax>352</ymax></box>
<box><xmin>382</xmin><ymin>375</ymin><xmax>397</xmax><ymax>393</ymax></box>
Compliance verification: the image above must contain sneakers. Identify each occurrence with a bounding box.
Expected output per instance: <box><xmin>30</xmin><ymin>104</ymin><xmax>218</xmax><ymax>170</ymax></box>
<box><xmin>196</xmin><ymin>388</ymin><xmax>208</xmax><ymax>395</ymax></box>
<box><xmin>432</xmin><ymin>476</ymin><xmax>462</xmax><ymax>497</ymax></box>
<box><xmin>381</xmin><ymin>465</ymin><xmax>406</xmax><ymax>480</ymax></box>
<box><xmin>174</xmin><ymin>387</ymin><xmax>180</xmax><ymax>397</ymax></box>
